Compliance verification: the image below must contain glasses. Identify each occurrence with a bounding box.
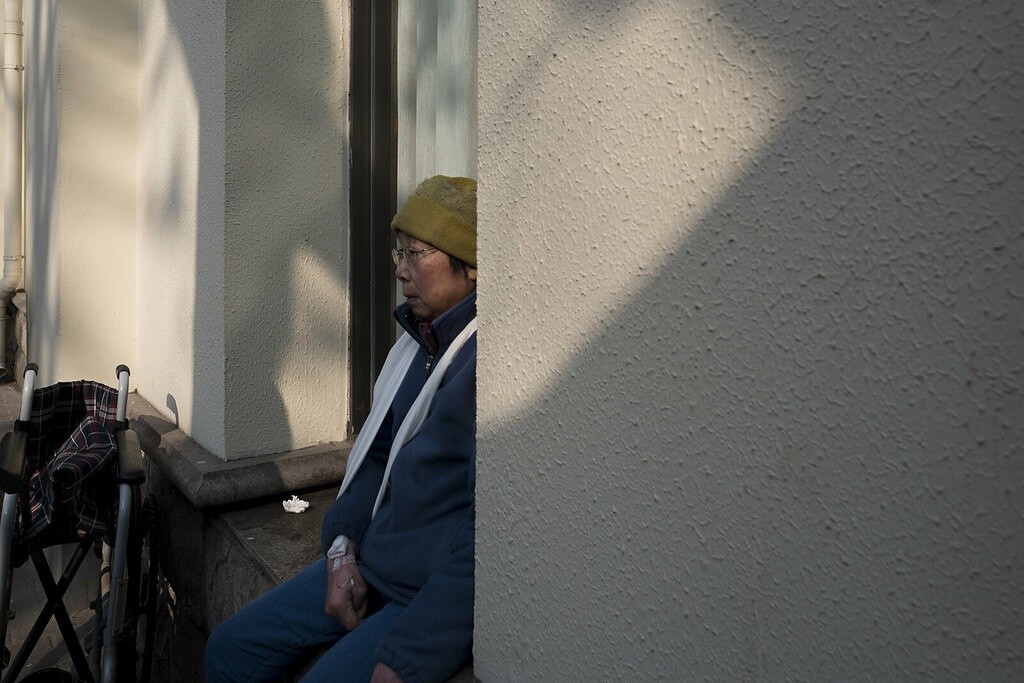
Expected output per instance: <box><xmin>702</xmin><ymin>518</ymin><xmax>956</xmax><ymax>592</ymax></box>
<box><xmin>391</xmin><ymin>246</ymin><xmax>435</xmax><ymax>266</ymax></box>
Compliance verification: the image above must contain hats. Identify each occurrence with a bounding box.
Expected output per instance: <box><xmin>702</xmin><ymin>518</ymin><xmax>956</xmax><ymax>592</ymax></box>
<box><xmin>391</xmin><ymin>174</ymin><xmax>477</xmax><ymax>268</ymax></box>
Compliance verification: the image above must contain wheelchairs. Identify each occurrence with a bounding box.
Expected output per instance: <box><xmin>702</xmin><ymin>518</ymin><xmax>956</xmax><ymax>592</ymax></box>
<box><xmin>0</xmin><ymin>361</ymin><xmax>149</xmax><ymax>682</ymax></box>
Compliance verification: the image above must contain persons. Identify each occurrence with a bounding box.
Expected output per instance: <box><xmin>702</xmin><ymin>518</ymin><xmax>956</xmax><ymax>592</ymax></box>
<box><xmin>205</xmin><ymin>175</ymin><xmax>476</xmax><ymax>683</ymax></box>
